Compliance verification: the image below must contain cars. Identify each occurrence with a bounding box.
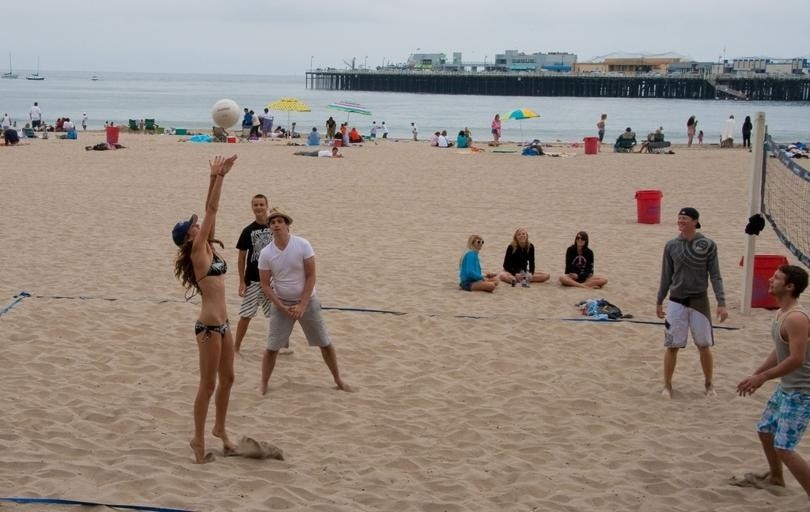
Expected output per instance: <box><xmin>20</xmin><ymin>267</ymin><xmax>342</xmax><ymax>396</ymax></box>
<box><xmin>685</xmin><ymin>71</ymin><xmax>698</xmax><ymax>79</ymax></box>
<box><xmin>648</xmin><ymin>71</ymin><xmax>663</xmax><ymax>77</ymax></box>
<box><xmin>667</xmin><ymin>71</ymin><xmax>681</xmax><ymax>78</ymax></box>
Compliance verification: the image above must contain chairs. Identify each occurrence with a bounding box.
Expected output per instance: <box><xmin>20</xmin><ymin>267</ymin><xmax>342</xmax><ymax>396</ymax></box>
<box><xmin>614</xmin><ymin>133</ymin><xmax>637</xmax><ymax>153</ymax></box>
<box><xmin>144</xmin><ymin>118</ymin><xmax>159</xmax><ymax>131</ymax></box>
<box><xmin>23</xmin><ymin>128</ymin><xmax>35</xmax><ymax>136</ymax></box>
<box><xmin>127</xmin><ymin>119</ymin><xmax>141</xmax><ymax>132</ymax></box>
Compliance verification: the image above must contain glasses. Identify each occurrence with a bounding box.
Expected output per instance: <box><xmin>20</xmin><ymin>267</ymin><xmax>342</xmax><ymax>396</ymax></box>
<box><xmin>477</xmin><ymin>240</ymin><xmax>485</xmax><ymax>244</ymax></box>
<box><xmin>575</xmin><ymin>237</ymin><xmax>585</xmax><ymax>240</ymax></box>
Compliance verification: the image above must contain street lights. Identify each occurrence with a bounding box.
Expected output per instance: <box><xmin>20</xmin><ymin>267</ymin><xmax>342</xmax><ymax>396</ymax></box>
<box><xmin>722</xmin><ymin>47</ymin><xmax>726</xmax><ymax>60</ymax></box>
<box><xmin>483</xmin><ymin>55</ymin><xmax>488</xmax><ymax>72</ymax></box>
<box><xmin>311</xmin><ymin>56</ymin><xmax>313</xmax><ymax>72</ymax></box>
<box><xmin>717</xmin><ymin>55</ymin><xmax>722</xmax><ymax>74</ymax></box>
<box><xmin>365</xmin><ymin>56</ymin><xmax>370</xmax><ymax>73</ymax></box>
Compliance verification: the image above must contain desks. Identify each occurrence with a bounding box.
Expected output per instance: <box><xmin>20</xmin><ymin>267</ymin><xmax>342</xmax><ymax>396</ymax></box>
<box><xmin>641</xmin><ymin>141</ymin><xmax>671</xmax><ymax>154</ymax></box>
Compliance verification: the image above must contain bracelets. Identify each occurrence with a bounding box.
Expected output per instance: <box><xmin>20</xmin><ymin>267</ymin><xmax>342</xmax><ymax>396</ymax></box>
<box><xmin>217</xmin><ymin>172</ymin><xmax>224</xmax><ymax>176</ymax></box>
<box><xmin>210</xmin><ymin>174</ymin><xmax>217</xmax><ymax>177</ymax></box>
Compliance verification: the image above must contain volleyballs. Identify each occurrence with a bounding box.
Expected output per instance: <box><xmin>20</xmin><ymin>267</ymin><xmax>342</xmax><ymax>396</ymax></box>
<box><xmin>212</xmin><ymin>99</ymin><xmax>241</xmax><ymax>128</ymax></box>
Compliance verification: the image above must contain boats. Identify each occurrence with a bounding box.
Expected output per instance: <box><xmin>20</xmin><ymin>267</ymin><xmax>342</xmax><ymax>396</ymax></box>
<box><xmin>91</xmin><ymin>75</ymin><xmax>99</xmax><ymax>81</ymax></box>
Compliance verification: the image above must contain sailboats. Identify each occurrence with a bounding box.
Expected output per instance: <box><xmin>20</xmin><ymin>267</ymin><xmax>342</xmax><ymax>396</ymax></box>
<box><xmin>2</xmin><ymin>52</ymin><xmax>19</xmax><ymax>78</ymax></box>
<box><xmin>25</xmin><ymin>55</ymin><xmax>45</xmax><ymax>80</ymax></box>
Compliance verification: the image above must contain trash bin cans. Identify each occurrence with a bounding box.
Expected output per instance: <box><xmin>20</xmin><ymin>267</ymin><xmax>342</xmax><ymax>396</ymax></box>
<box><xmin>107</xmin><ymin>127</ymin><xmax>120</xmax><ymax>144</ymax></box>
<box><xmin>635</xmin><ymin>190</ymin><xmax>663</xmax><ymax>224</ymax></box>
<box><xmin>740</xmin><ymin>254</ymin><xmax>788</xmax><ymax>308</ymax></box>
<box><xmin>583</xmin><ymin>136</ymin><xmax>599</xmax><ymax>154</ymax></box>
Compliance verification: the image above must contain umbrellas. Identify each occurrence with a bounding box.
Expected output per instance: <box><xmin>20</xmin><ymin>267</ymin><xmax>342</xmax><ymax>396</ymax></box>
<box><xmin>327</xmin><ymin>99</ymin><xmax>372</xmax><ymax>122</ymax></box>
<box><xmin>265</xmin><ymin>98</ymin><xmax>311</xmax><ymax>129</ymax></box>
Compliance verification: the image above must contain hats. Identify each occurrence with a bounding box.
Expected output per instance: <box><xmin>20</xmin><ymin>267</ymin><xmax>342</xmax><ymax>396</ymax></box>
<box><xmin>171</xmin><ymin>214</ymin><xmax>198</xmax><ymax>245</ymax></box>
<box><xmin>678</xmin><ymin>207</ymin><xmax>701</xmax><ymax>229</ymax></box>
<box><xmin>263</xmin><ymin>206</ymin><xmax>293</xmax><ymax>225</ymax></box>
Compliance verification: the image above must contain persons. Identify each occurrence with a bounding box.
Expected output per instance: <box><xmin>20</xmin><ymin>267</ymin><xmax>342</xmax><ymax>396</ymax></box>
<box><xmin>735</xmin><ymin>265</ymin><xmax>810</xmax><ymax>498</ymax></box>
<box><xmin>234</xmin><ymin>194</ymin><xmax>274</xmax><ymax>358</ymax></box>
<box><xmin>498</xmin><ymin>227</ymin><xmax>551</xmax><ymax>287</ymax></box>
<box><xmin>172</xmin><ymin>153</ymin><xmax>239</xmax><ymax>464</ymax></box>
<box><xmin>559</xmin><ymin>231</ymin><xmax>608</xmax><ymax>289</ymax></box>
<box><xmin>255</xmin><ymin>207</ymin><xmax>353</xmax><ymax>396</ymax></box>
<box><xmin>458</xmin><ymin>235</ymin><xmax>500</xmax><ymax>292</ymax></box>
<box><xmin>656</xmin><ymin>207</ymin><xmax>729</xmax><ymax>400</ymax></box>
<box><xmin>0</xmin><ymin>103</ymin><xmax>756</xmax><ymax>156</ymax></box>
